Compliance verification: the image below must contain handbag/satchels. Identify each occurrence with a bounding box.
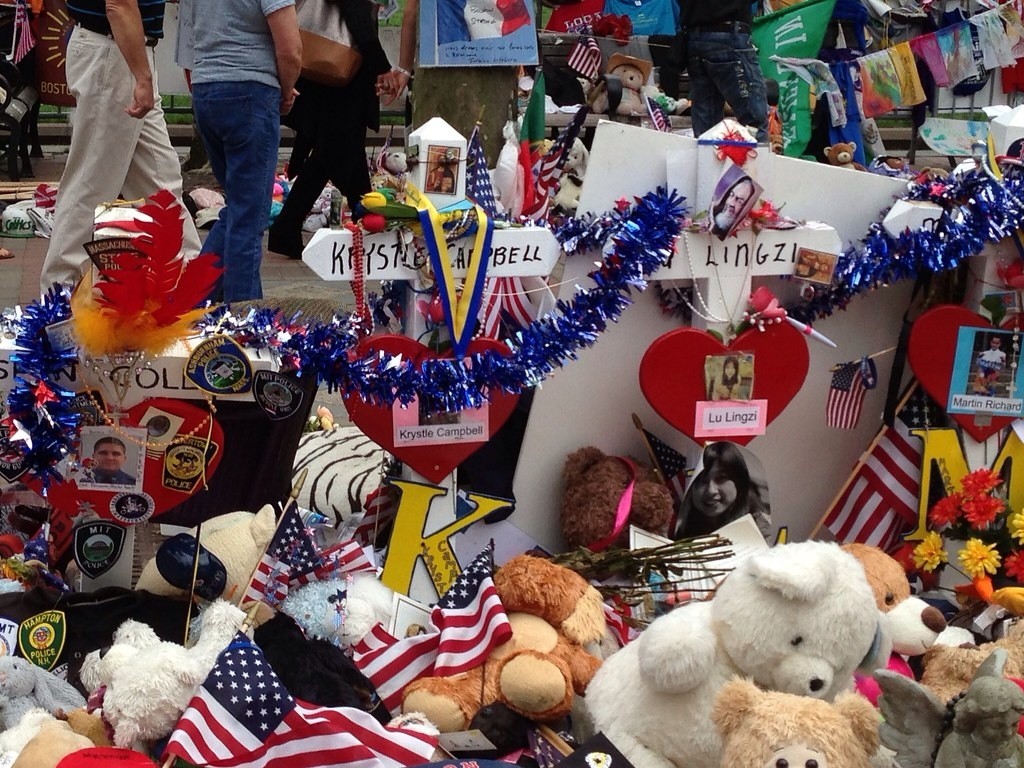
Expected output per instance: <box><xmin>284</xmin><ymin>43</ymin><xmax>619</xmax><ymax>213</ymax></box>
<box><xmin>297</xmin><ymin>0</ymin><xmax>364</xmax><ymax>88</ymax></box>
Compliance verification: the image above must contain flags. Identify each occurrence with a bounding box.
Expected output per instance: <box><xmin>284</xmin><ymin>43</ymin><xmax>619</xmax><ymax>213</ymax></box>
<box><xmin>858</xmin><ymin>418</ymin><xmax>924</xmax><ymax>528</ymax></box>
<box><xmin>824</xmin><ymin>472</ymin><xmax>909</xmax><ymax>559</ymax></box>
<box><xmin>518</xmin><ymin>66</ymin><xmax>603</xmax><ymax>224</ymax></box>
<box><xmin>376</xmin><ymin>130</ymin><xmax>393</xmax><ymax>175</ymax></box>
<box><xmin>15</xmin><ymin>0</ymin><xmax>36</xmax><ymax>64</ymax></box>
<box><xmin>245</xmin><ymin>501</ymin><xmax>318</xmax><ymax>608</ymax></box>
<box><xmin>353</xmin><ymin>540</ymin><xmax>513</xmax><ymax>711</ymax></box>
<box><xmin>753</xmin><ymin>0</ymin><xmax>1024</xmax><ymax>128</ymax></box>
<box><xmin>465</xmin><ymin>106</ymin><xmax>588</xmax><ymax>340</ymax></box>
<box><xmin>435</xmin><ymin>0</ymin><xmax>532</xmax><ymax>65</ymax></box>
<box><xmin>825</xmin><ymin>362</ymin><xmax>867</xmax><ymax>430</ymax></box>
<box><xmin>159</xmin><ymin>630</ymin><xmax>439</xmax><ymax>768</ymax></box>
<box><xmin>568</xmin><ymin>27</ymin><xmax>602</xmax><ymax>85</ymax></box>
<box><xmin>264</xmin><ymin>539</ymin><xmax>377</xmax><ymax>613</ymax></box>
<box><xmin>646</xmin><ymin>96</ymin><xmax>673</xmax><ymax>133</ymax></box>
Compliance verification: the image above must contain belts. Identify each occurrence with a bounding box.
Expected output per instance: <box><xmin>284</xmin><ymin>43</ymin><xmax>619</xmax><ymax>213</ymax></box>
<box><xmin>75</xmin><ymin>19</ymin><xmax>158</xmax><ymax>48</ymax></box>
<box><xmin>688</xmin><ymin>21</ymin><xmax>752</xmax><ymax>34</ymax></box>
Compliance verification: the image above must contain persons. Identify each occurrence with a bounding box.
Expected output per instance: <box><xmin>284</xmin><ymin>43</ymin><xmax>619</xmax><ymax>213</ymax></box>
<box><xmin>674</xmin><ymin>442</ymin><xmax>768</xmax><ymax>541</ymax></box>
<box><xmin>794</xmin><ymin>250</ymin><xmax>830</xmax><ymax>279</ymax></box>
<box><xmin>146</xmin><ymin>416</ymin><xmax>170</xmax><ymax>438</ymax></box>
<box><xmin>713</xmin><ymin>357</ymin><xmax>741</xmax><ymax>401</ymax></box>
<box><xmin>426</xmin><ymin>154</ymin><xmax>454</xmax><ymax>193</ymax></box>
<box><xmin>673</xmin><ymin>0</ymin><xmax>769</xmax><ymax>143</ymax></box>
<box><xmin>935</xmin><ymin>676</ymin><xmax>1023</xmax><ymax>768</ymax></box>
<box><xmin>37</xmin><ymin>0</ymin><xmax>535</xmax><ymax>310</ymax></box>
<box><xmin>974</xmin><ymin>336</ymin><xmax>1006</xmax><ymax>398</ymax></box>
<box><xmin>705</xmin><ymin>175</ymin><xmax>755</xmax><ymax>241</ymax></box>
<box><xmin>78</xmin><ymin>437</ymin><xmax>136</xmax><ymax>486</ymax></box>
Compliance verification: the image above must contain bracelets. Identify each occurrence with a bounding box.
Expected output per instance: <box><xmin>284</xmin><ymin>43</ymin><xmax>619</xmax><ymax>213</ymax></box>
<box><xmin>396</xmin><ymin>65</ymin><xmax>411</xmax><ymax>78</ymax></box>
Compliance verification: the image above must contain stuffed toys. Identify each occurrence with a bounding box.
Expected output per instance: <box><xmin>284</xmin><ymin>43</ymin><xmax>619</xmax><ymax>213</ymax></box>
<box><xmin>555</xmin><ymin>137</ymin><xmax>589</xmax><ymax>210</ymax></box>
<box><xmin>587</xmin><ymin>51</ymin><xmax>646</xmax><ymax>115</ymax></box>
<box><xmin>386</xmin><ymin>151</ymin><xmax>408</xmax><ymax>175</ymax></box>
<box><xmin>868</xmin><ymin>155</ymin><xmax>949</xmax><ymax>184</ymax></box>
<box><xmin>537</xmin><ymin>138</ymin><xmax>555</xmax><ymax>154</ymax></box>
<box><xmin>823</xmin><ymin>142</ymin><xmax>867</xmax><ymax>172</ymax></box>
<box><xmin>189</xmin><ymin>170</ymin><xmax>350</xmax><ymax>233</ymax></box>
<box><xmin>1</xmin><ymin>404</ymin><xmax>1024</xmax><ymax>768</ymax></box>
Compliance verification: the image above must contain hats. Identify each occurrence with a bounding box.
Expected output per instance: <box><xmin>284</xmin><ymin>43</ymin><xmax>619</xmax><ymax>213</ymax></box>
<box><xmin>0</xmin><ymin>183</ymin><xmax>58</xmax><ymax>238</ymax></box>
<box><xmin>182</xmin><ymin>188</ymin><xmax>229</xmax><ymax>228</ymax></box>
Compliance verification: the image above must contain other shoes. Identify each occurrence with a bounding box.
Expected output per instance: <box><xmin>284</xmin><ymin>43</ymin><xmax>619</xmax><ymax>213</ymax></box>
<box><xmin>0</xmin><ymin>247</ymin><xmax>15</xmax><ymax>260</ymax></box>
<box><xmin>267</xmin><ymin>232</ymin><xmax>306</xmax><ymax>257</ymax></box>
